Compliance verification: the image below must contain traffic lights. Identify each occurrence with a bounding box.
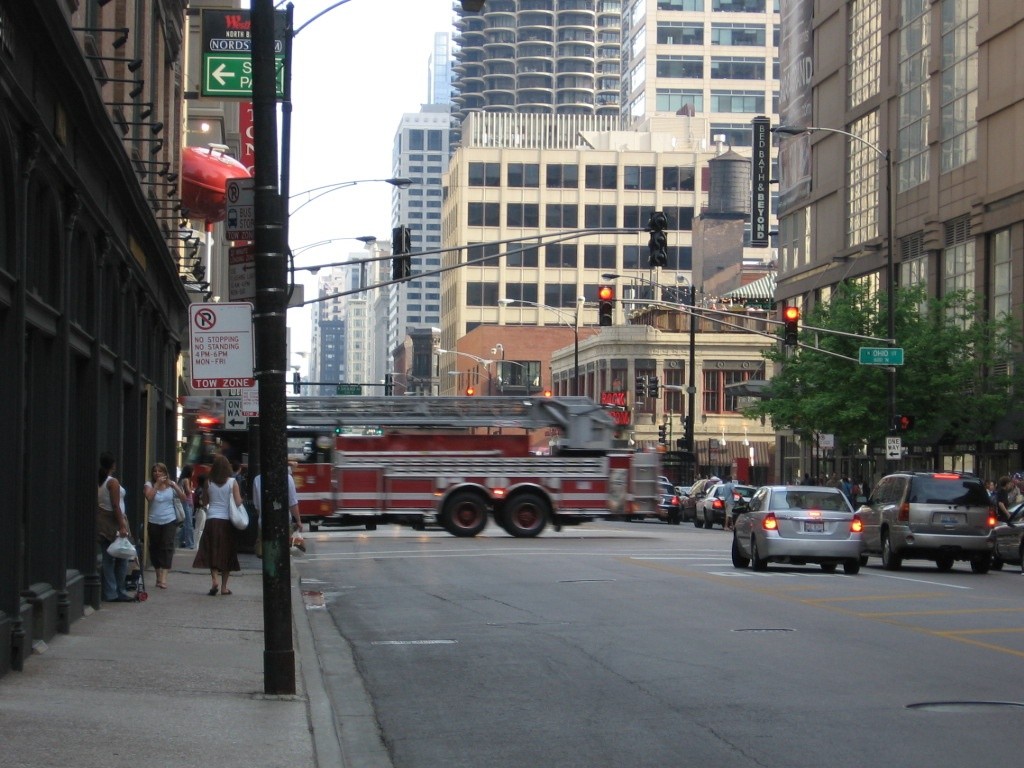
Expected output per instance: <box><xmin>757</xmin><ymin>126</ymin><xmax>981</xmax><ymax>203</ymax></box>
<box><xmin>658</xmin><ymin>425</ymin><xmax>665</xmax><ymax>442</ymax></box>
<box><xmin>898</xmin><ymin>414</ymin><xmax>915</xmax><ymax>430</ymax></box>
<box><xmin>783</xmin><ymin>306</ymin><xmax>796</xmax><ymax>343</ymax></box>
<box><xmin>596</xmin><ymin>284</ymin><xmax>613</xmax><ymax>326</ymax></box>
<box><xmin>676</xmin><ymin>438</ymin><xmax>688</xmax><ymax>449</ymax></box>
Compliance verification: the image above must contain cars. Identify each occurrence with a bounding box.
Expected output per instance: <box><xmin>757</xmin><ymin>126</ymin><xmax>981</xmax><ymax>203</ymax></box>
<box><xmin>851</xmin><ymin>469</ymin><xmax>997</xmax><ymax>575</ymax></box>
<box><xmin>657</xmin><ymin>475</ymin><xmax>754</xmax><ymax>530</ymax></box>
<box><xmin>995</xmin><ymin>503</ymin><xmax>1024</xmax><ymax>575</ymax></box>
<box><xmin>733</xmin><ymin>486</ymin><xmax>861</xmax><ymax>571</ymax></box>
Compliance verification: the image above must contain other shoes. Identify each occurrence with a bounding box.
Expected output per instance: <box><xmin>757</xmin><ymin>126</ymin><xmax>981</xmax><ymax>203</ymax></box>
<box><xmin>155</xmin><ymin>580</ymin><xmax>167</xmax><ymax>589</ymax></box>
<box><xmin>222</xmin><ymin>590</ymin><xmax>232</xmax><ymax>595</ymax></box>
<box><xmin>209</xmin><ymin>589</ymin><xmax>218</xmax><ymax>595</ymax></box>
<box><xmin>103</xmin><ymin>596</ymin><xmax>132</xmax><ymax>602</ymax></box>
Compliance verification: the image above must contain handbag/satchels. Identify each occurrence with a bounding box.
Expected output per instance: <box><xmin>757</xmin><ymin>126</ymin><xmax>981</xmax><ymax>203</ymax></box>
<box><xmin>107</xmin><ymin>537</ymin><xmax>136</xmax><ymax>560</ymax></box>
<box><xmin>739</xmin><ymin>498</ymin><xmax>744</xmax><ymax>505</ymax></box>
<box><xmin>174</xmin><ymin>493</ymin><xmax>185</xmax><ymax>523</ymax></box>
<box><xmin>288</xmin><ymin>528</ymin><xmax>306</xmax><ymax>556</ymax></box>
<box><xmin>230</xmin><ymin>485</ymin><xmax>249</xmax><ymax>530</ymax></box>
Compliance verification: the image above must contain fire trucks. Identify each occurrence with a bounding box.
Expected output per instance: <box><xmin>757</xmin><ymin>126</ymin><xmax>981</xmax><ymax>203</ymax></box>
<box><xmin>287</xmin><ymin>418</ymin><xmax>664</xmax><ymax>540</ymax></box>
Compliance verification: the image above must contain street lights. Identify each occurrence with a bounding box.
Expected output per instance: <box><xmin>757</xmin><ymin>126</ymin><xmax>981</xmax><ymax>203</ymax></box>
<box><xmin>601</xmin><ymin>270</ymin><xmax>682</xmax><ymax>305</ymax></box>
<box><xmin>436</xmin><ymin>347</ymin><xmax>492</xmax><ymax>397</ymax></box>
<box><xmin>499</xmin><ymin>296</ymin><xmax>580</xmax><ymax>395</ymax></box>
<box><xmin>771</xmin><ymin>117</ymin><xmax>898</xmax><ymax>475</ymax></box>
<box><xmin>288</xmin><ymin>174</ymin><xmax>424</xmax><ymax>217</ymax></box>
<box><xmin>289</xmin><ymin>234</ymin><xmax>376</xmax><ymax>255</ymax></box>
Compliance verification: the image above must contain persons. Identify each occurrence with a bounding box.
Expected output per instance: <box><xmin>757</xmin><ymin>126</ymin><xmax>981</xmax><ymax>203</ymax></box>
<box><xmin>722</xmin><ymin>476</ymin><xmax>742</xmax><ymax>530</ymax></box>
<box><xmin>252</xmin><ymin>472</ymin><xmax>306</xmax><ymax>559</ymax></box>
<box><xmin>799</xmin><ymin>471</ymin><xmax>867</xmax><ymax>511</ymax></box>
<box><xmin>145</xmin><ymin>457</ymin><xmax>259</xmax><ymax>596</ymax></box>
<box><xmin>97</xmin><ymin>451</ymin><xmax>138</xmax><ymax>602</ymax></box>
<box><xmin>986</xmin><ymin>473</ymin><xmax>1024</xmax><ymax>522</ymax></box>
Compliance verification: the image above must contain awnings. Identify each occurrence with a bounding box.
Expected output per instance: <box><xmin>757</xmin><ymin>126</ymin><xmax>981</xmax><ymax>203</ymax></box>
<box><xmin>629</xmin><ymin>440</ymin><xmax>769</xmax><ymax>467</ymax></box>
